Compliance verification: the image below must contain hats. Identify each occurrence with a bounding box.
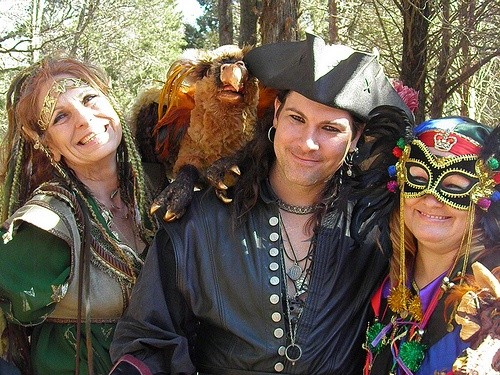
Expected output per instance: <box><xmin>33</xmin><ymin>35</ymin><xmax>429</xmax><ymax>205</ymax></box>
<box><xmin>242</xmin><ymin>32</ymin><xmax>415</xmax><ymax>140</ymax></box>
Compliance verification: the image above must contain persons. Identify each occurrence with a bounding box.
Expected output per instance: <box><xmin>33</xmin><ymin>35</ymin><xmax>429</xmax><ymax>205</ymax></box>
<box><xmin>1</xmin><ymin>58</ymin><xmax>166</xmax><ymax>375</ymax></box>
<box><xmin>111</xmin><ymin>32</ymin><xmax>413</xmax><ymax>375</ymax></box>
<box><xmin>360</xmin><ymin>117</ymin><xmax>500</xmax><ymax>374</ymax></box>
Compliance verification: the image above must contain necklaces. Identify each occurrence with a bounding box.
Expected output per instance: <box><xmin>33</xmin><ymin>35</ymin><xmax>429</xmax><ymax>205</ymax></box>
<box><xmin>278</xmin><ymin>212</ymin><xmax>313</xmax><ymax>362</ymax></box>
<box><xmin>101</xmin><ymin>202</ymin><xmax>129</xmax><ymax>221</ymax></box>
<box><xmin>270</xmin><ymin>188</ymin><xmax>317</xmax><ymax>215</ymax></box>
